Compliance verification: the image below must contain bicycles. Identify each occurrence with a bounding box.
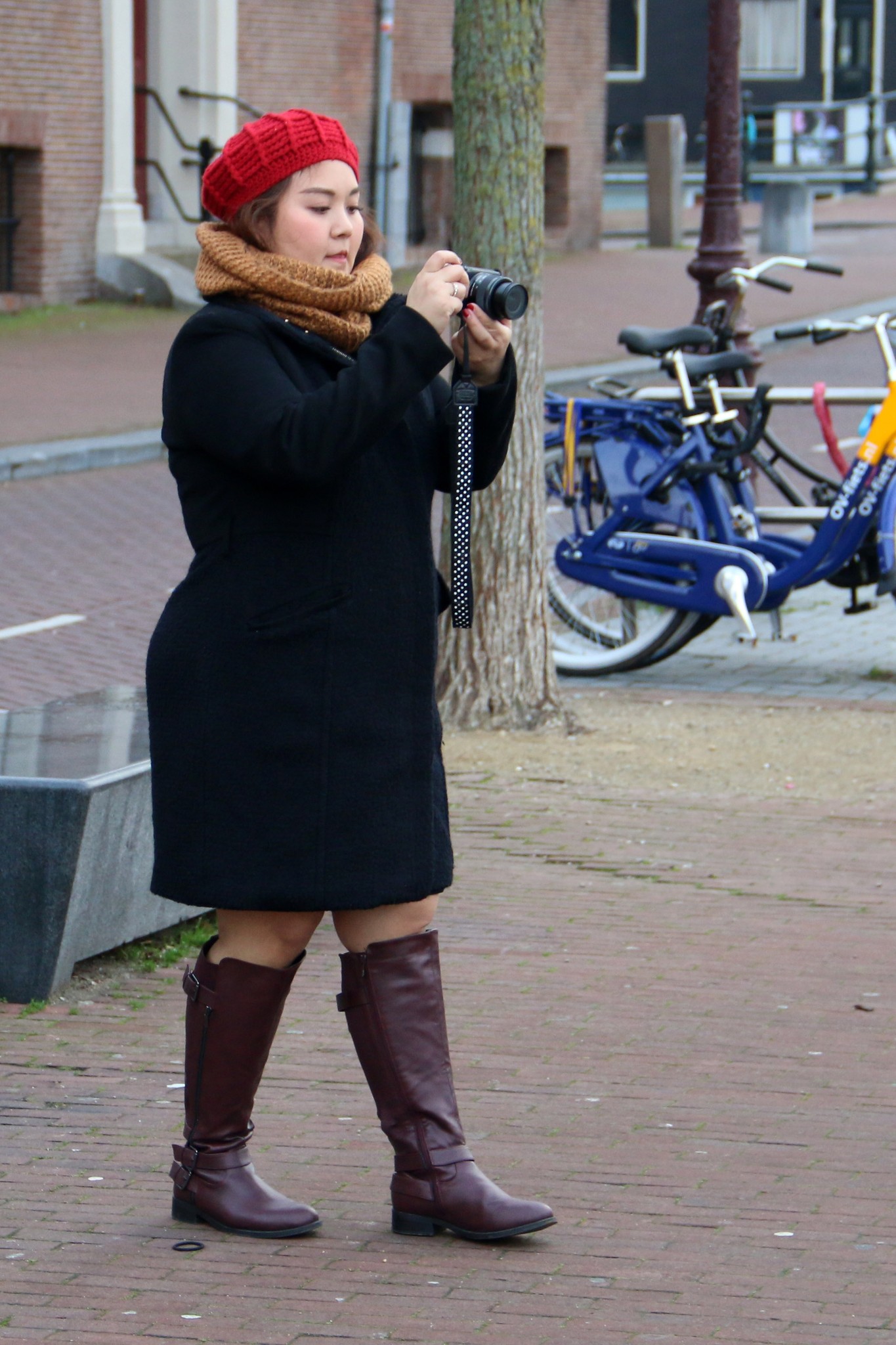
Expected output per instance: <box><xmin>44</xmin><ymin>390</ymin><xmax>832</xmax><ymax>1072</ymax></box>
<box><xmin>540</xmin><ymin>252</ymin><xmax>896</xmax><ymax>680</ymax></box>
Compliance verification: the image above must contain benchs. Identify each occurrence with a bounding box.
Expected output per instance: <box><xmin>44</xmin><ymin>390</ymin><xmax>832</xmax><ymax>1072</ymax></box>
<box><xmin>0</xmin><ymin>679</ymin><xmax>217</xmax><ymax>1004</ymax></box>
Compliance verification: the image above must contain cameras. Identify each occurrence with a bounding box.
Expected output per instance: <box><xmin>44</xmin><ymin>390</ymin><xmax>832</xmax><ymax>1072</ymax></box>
<box><xmin>457</xmin><ymin>265</ymin><xmax>529</xmax><ymax>321</ymax></box>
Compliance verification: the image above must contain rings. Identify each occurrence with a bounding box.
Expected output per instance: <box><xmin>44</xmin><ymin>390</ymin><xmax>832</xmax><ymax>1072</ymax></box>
<box><xmin>451</xmin><ymin>282</ymin><xmax>460</xmax><ymax>298</ymax></box>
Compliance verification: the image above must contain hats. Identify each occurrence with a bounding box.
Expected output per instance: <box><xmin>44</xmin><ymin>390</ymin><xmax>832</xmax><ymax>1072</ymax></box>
<box><xmin>200</xmin><ymin>108</ymin><xmax>359</xmax><ymax>227</ymax></box>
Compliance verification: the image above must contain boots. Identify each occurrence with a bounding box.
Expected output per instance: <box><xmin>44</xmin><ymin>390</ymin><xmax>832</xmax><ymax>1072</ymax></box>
<box><xmin>335</xmin><ymin>928</ymin><xmax>559</xmax><ymax>1240</ymax></box>
<box><xmin>168</xmin><ymin>935</ymin><xmax>322</xmax><ymax>1238</ymax></box>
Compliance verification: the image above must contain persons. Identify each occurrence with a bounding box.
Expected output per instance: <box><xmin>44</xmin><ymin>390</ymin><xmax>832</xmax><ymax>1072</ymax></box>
<box><xmin>145</xmin><ymin>108</ymin><xmax>555</xmax><ymax>1238</ymax></box>
